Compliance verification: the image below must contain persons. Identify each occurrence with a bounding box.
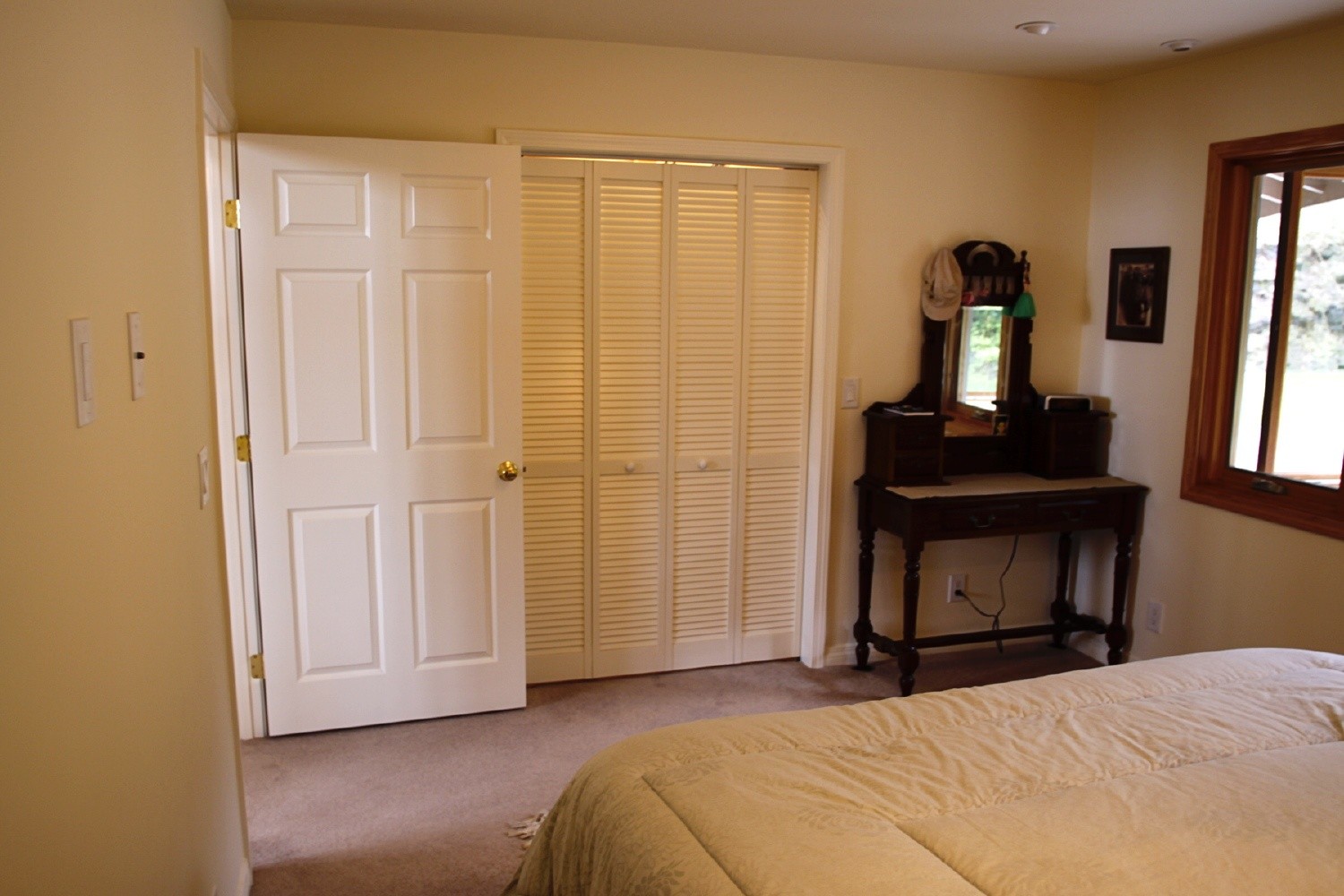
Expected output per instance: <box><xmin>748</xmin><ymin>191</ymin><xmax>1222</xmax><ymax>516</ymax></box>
<box><xmin>1117</xmin><ymin>272</ymin><xmax>1151</xmax><ymax>328</ymax></box>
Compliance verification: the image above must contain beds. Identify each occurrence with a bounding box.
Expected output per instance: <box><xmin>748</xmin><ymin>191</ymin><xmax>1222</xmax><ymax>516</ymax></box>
<box><xmin>504</xmin><ymin>646</ymin><xmax>1344</xmax><ymax>896</ymax></box>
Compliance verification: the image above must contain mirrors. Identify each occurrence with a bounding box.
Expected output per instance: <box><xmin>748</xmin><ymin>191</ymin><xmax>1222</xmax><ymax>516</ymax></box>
<box><xmin>918</xmin><ymin>240</ymin><xmax>1032</xmax><ymax>477</ymax></box>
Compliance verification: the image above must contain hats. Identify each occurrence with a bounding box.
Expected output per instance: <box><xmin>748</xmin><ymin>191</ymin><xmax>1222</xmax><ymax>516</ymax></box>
<box><xmin>921</xmin><ymin>247</ymin><xmax>964</xmax><ymax>320</ymax></box>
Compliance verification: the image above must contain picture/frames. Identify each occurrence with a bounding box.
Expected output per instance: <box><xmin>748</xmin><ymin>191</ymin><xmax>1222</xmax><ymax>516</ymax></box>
<box><xmin>1105</xmin><ymin>245</ymin><xmax>1170</xmax><ymax>344</ymax></box>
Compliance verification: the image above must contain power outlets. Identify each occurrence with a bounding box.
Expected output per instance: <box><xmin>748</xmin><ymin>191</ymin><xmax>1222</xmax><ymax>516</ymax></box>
<box><xmin>1148</xmin><ymin>600</ymin><xmax>1164</xmax><ymax>632</ymax></box>
<box><xmin>948</xmin><ymin>574</ymin><xmax>965</xmax><ymax>602</ymax></box>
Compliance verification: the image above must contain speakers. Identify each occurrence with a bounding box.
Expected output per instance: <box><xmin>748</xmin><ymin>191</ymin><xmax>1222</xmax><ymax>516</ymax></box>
<box><xmin>1013</xmin><ymin>409</ymin><xmax>1105</xmax><ymax>479</ymax></box>
<box><xmin>870</xmin><ymin>411</ymin><xmax>945</xmax><ymax>483</ymax></box>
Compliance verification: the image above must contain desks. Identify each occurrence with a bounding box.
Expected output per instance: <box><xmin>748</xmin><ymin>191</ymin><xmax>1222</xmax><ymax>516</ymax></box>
<box><xmin>854</xmin><ymin>473</ymin><xmax>1150</xmax><ymax>696</ymax></box>
